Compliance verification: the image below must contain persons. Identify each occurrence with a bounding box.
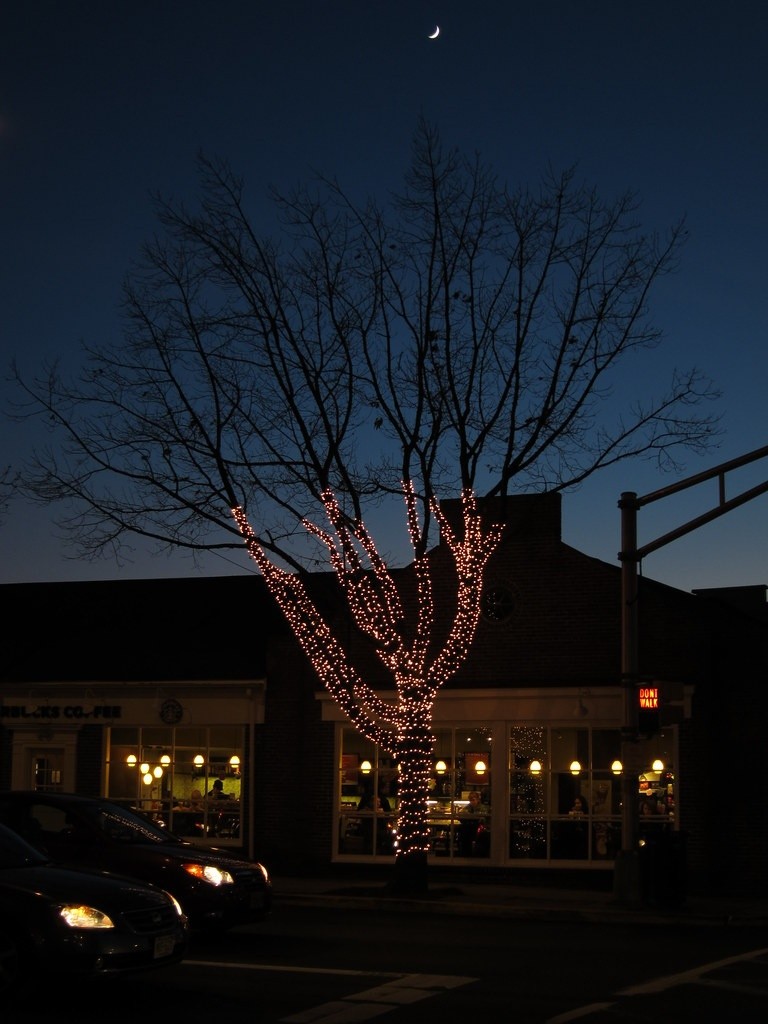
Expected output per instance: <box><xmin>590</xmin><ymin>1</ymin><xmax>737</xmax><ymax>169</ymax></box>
<box><xmin>639</xmin><ymin>800</ymin><xmax>667</xmax><ymax>848</ymax></box>
<box><xmin>357</xmin><ymin>792</ymin><xmax>394</xmax><ymax>855</ymax></box>
<box><xmin>569</xmin><ymin>796</ymin><xmax>588</xmax><ymax>815</ymax></box>
<box><xmin>460</xmin><ymin>787</ymin><xmax>491</xmax><ymax>827</ymax></box>
<box><xmin>162</xmin><ymin>792</ymin><xmax>178</xmax><ymax>824</ymax></box>
<box><xmin>202</xmin><ymin>780</ymin><xmax>228</xmax><ymax>800</ymax></box>
<box><xmin>192</xmin><ymin>790</ymin><xmax>201</xmax><ymax>806</ymax></box>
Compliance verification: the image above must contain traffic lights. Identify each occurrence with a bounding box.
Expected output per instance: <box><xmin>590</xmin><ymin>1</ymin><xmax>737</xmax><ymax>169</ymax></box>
<box><xmin>636</xmin><ymin>686</ymin><xmax>662</xmax><ymax>741</ymax></box>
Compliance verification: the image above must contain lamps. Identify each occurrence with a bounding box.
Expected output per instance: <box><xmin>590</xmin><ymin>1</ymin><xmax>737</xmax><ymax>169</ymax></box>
<box><xmin>81</xmin><ymin>689</ymin><xmax>105</xmax><ymax>714</ymax></box>
<box><xmin>25</xmin><ymin>689</ymin><xmax>48</xmax><ymax>714</ymax></box>
<box><xmin>573</xmin><ymin>686</ymin><xmax>592</xmax><ymax>718</ymax></box>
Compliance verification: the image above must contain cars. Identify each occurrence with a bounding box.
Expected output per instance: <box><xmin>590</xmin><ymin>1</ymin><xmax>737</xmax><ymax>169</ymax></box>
<box><xmin>0</xmin><ymin>823</ymin><xmax>190</xmax><ymax>990</ymax></box>
<box><xmin>0</xmin><ymin>788</ymin><xmax>273</xmax><ymax>934</ymax></box>
